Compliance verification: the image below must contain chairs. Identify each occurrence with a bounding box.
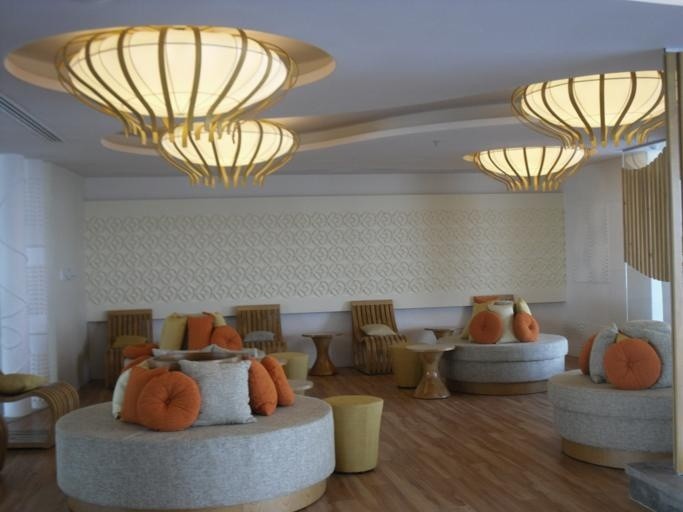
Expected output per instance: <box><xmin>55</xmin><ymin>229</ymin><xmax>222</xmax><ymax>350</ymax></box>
<box><xmin>234</xmin><ymin>304</ymin><xmax>287</xmax><ymax>352</ymax></box>
<box><xmin>352</xmin><ymin>299</ymin><xmax>405</xmax><ymax>375</ymax></box>
<box><xmin>0</xmin><ymin>371</ymin><xmax>79</xmax><ymax>449</ymax></box>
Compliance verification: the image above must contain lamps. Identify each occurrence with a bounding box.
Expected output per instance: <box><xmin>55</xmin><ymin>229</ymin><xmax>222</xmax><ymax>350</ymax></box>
<box><xmin>158</xmin><ymin>115</ymin><xmax>299</xmax><ymax>190</ymax></box>
<box><xmin>511</xmin><ymin>70</ymin><xmax>667</xmax><ymax>150</ymax></box>
<box><xmin>474</xmin><ymin>146</ymin><xmax>586</xmax><ymax>192</ymax></box>
<box><xmin>53</xmin><ymin>24</ymin><xmax>299</xmax><ymax>148</ymax></box>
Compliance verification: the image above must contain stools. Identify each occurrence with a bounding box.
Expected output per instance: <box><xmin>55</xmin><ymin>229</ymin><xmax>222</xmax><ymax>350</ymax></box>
<box><xmin>321</xmin><ymin>394</ymin><xmax>384</xmax><ymax>474</ymax></box>
<box><xmin>270</xmin><ymin>352</ymin><xmax>308</xmax><ymax>380</ymax></box>
<box><xmin>389</xmin><ymin>341</ymin><xmax>422</xmax><ymax>388</ymax></box>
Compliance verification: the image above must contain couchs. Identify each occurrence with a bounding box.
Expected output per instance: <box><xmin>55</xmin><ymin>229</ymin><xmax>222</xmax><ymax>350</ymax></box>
<box><xmin>449</xmin><ymin>332</ymin><xmax>569</xmax><ymax>395</ymax></box>
<box><xmin>54</xmin><ymin>402</ymin><xmax>335</xmax><ymax>511</ymax></box>
<box><xmin>547</xmin><ymin>369</ymin><xmax>674</xmax><ymax>470</ymax></box>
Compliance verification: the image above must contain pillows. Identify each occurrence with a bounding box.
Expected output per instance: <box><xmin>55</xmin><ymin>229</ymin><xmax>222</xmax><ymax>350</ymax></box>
<box><xmin>112</xmin><ymin>312</ymin><xmax>297</xmax><ymax>432</ymax></box>
<box><xmin>460</xmin><ymin>293</ymin><xmax>540</xmax><ymax>343</ymax></box>
<box><xmin>0</xmin><ymin>374</ymin><xmax>48</xmax><ymax>394</ymax></box>
<box><xmin>360</xmin><ymin>323</ymin><xmax>396</xmax><ymax>336</ymax></box>
<box><xmin>578</xmin><ymin>318</ymin><xmax>674</xmax><ymax>389</ymax></box>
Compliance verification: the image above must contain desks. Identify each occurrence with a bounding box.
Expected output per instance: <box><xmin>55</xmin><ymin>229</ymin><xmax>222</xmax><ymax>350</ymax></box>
<box><xmin>302</xmin><ymin>331</ymin><xmax>346</xmax><ymax>376</ymax></box>
<box><xmin>289</xmin><ymin>378</ymin><xmax>315</xmax><ymax>396</ymax></box>
<box><xmin>407</xmin><ymin>345</ymin><xmax>457</xmax><ymax>399</ymax></box>
